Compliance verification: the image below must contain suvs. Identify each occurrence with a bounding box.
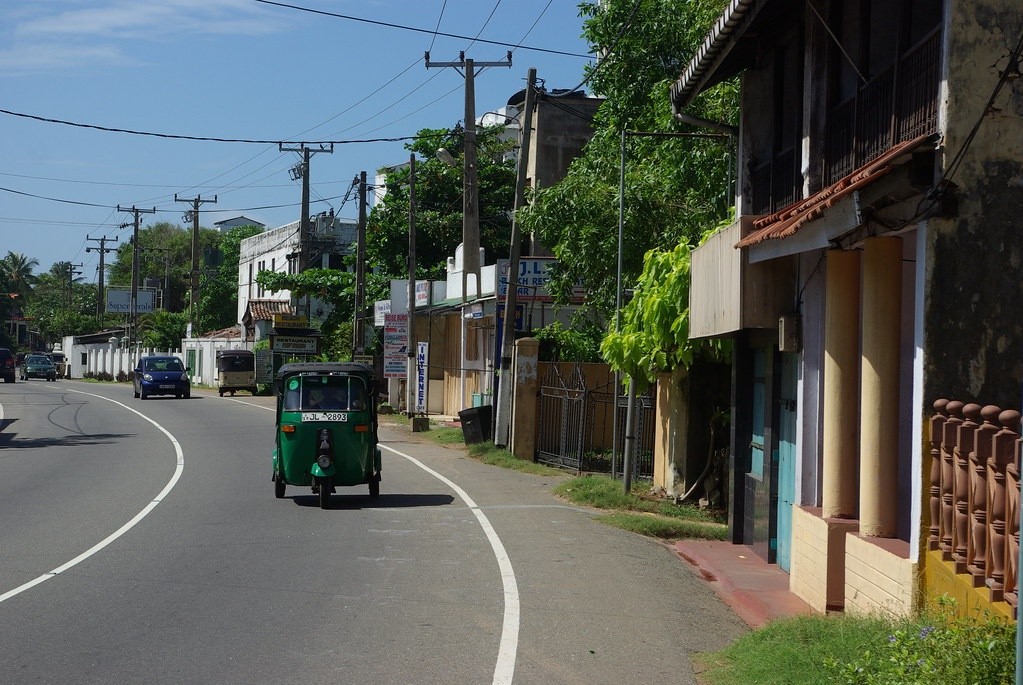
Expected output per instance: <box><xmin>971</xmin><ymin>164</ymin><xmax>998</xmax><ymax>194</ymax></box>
<box><xmin>0</xmin><ymin>348</ymin><xmax>16</xmax><ymax>383</ymax></box>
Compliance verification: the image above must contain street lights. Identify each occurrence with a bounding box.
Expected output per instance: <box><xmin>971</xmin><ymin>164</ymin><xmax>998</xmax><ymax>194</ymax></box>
<box><xmin>477</xmin><ymin>112</ymin><xmax>529</xmax><ymax>447</ymax></box>
<box><xmin>435</xmin><ymin>146</ymin><xmax>484</xmax><ymax>409</ymax></box>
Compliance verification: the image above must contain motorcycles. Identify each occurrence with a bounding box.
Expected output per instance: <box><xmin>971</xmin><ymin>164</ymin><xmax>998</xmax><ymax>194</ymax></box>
<box><xmin>32</xmin><ymin>352</ymin><xmax>68</xmax><ymax>379</ymax></box>
<box><xmin>272</xmin><ymin>362</ymin><xmax>382</xmax><ymax>510</ymax></box>
<box><xmin>216</xmin><ymin>351</ymin><xmax>259</xmax><ymax>399</ymax></box>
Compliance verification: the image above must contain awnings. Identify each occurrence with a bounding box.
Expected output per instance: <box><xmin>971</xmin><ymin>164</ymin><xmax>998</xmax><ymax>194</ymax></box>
<box><xmin>415</xmin><ymin>292</ymin><xmax>495</xmax><ymax>308</ymax></box>
<box><xmin>733</xmin><ymin>134</ymin><xmax>925</xmax><ymax>250</ymax></box>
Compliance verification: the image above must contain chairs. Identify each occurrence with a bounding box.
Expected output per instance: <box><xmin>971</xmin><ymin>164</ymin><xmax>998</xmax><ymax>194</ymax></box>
<box><xmin>147</xmin><ymin>363</ymin><xmax>157</xmax><ymax>370</ymax></box>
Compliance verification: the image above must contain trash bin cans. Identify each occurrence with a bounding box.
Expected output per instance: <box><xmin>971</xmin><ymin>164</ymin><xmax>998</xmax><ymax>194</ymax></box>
<box><xmin>458</xmin><ymin>405</ymin><xmax>492</xmax><ymax>446</ymax></box>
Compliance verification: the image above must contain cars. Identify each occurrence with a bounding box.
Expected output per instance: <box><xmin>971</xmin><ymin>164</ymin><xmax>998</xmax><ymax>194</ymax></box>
<box><xmin>20</xmin><ymin>355</ymin><xmax>56</xmax><ymax>382</ymax></box>
<box><xmin>132</xmin><ymin>355</ymin><xmax>192</xmax><ymax>399</ymax></box>
